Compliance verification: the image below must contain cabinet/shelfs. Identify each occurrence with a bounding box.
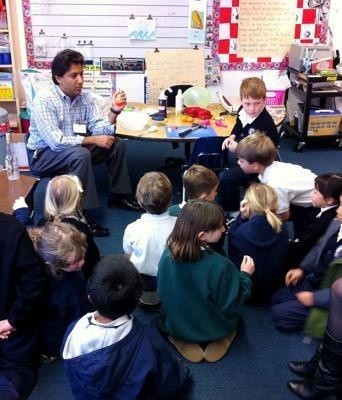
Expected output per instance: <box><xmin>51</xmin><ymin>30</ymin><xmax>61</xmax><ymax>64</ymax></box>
<box><xmin>0</xmin><ymin>0</ymin><xmax>30</xmax><ymax>130</ymax></box>
<box><xmin>282</xmin><ymin>63</ymin><xmax>342</xmax><ymax>152</ymax></box>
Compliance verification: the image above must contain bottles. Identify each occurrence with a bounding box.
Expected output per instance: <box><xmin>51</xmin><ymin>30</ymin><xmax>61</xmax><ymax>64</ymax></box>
<box><xmin>175</xmin><ymin>89</ymin><xmax>185</xmax><ymax>113</ymax></box>
<box><xmin>4</xmin><ymin>131</ymin><xmax>19</xmax><ymax>182</ymax></box>
<box><xmin>115</xmin><ymin>89</ymin><xmax>125</xmax><ymax>106</ymax></box>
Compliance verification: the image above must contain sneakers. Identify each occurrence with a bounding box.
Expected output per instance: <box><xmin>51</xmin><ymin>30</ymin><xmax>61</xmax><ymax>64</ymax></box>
<box><xmin>168</xmin><ymin>335</ymin><xmax>204</xmax><ymax>362</ymax></box>
<box><xmin>139</xmin><ymin>291</ymin><xmax>161</xmax><ymax>312</ymax></box>
<box><xmin>204</xmin><ymin>329</ymin><xmax>237</xmax><ymax>362</ymax></box>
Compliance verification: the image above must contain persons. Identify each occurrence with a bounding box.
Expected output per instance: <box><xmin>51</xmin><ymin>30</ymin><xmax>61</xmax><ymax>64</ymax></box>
<box><xmin>25</xmin><ymin>47</ymin><xmax>128</xmax><ymax>239</ymax></box>
<box><xmin>234</xmin><ymin>132</ymin><xmax>318</xmax><ymax>239</ymax></box>
<box><xmin>122</xmin><ymin>163</ymin><xmax>297</xmax><ymax>363</ymax></box>
<box><xmin>0</xmin><ymin>211</ymin><xmax>53</xmax><ymax>400</ymax></box>
<box><xmin>220</xmin><ymin>77</ymin><xmax>281</xmax><ymax>164</ymax></box>
<box><xmin>59</xmin><ymin>254</ymin><xmax>193</xmax><ymax>399</ymax></box>
<box><xmin>271</xmin><ymin>172</ymin><xmax>342</xmax><ymax>399</ymax></box>
<box><xmin>13</xmin><ymin>175</ymin><xmax>101</xmax><ymax>364</ymax></box>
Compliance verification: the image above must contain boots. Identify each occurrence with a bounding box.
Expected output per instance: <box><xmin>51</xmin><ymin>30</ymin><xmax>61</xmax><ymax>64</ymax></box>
<box><xmin>288</xmin><ymin>339</ymin><xmax>323</xmax><ymax>377</ymax></box>
<box><xmin>287</xmin><ymin>332</ymin><xmax>342</xmax><ymax>399</ymax></box>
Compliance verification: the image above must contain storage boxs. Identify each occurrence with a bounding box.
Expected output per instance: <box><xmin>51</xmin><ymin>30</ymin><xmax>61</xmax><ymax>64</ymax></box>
<box><xmin>8</xmin><ymin>133</ymin><xmax>32</xmax><ymax>172</ymax></box>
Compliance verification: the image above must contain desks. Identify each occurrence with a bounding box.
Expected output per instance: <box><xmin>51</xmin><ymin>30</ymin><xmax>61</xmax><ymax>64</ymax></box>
<box><xmin>106</xmin><ymin>105</ymin><xmax>285</xmax><ymax>175</ymax></box>
<box><xmin>0</xmin><ymin>169</ymin><xmax>37</xmax><ymax>216</ymax></box>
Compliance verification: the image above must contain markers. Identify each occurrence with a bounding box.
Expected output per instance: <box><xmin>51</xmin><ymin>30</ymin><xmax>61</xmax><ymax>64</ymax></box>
<box><xmin>179</xmin><ymin>126</ymin><xmax>199</xmax><ymax>137</ymax></box>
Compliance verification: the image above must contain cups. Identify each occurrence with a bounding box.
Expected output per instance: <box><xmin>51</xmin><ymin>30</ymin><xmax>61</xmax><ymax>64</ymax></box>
<box><xmin>337</xmin><ymin>66</ymin><xmax>342</xmax><ymax>76</ymax></box>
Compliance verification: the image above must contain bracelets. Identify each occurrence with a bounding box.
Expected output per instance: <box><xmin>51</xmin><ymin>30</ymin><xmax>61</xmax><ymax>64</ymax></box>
<box><xmin>109</xmin><ymin>107</ymin><xmax>122</xmax><ymax>115</ymax></box>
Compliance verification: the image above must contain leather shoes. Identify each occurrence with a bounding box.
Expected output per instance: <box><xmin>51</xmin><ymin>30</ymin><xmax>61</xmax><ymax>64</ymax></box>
<box><xmin>107</xmin><ymin>194</ymin><xmax>143</xmax><ymax>211</ymax></box>
<box><xmin>85</xmin><ymin>215</ymin><xmax>109</xmax><ymax>237</ymax></box>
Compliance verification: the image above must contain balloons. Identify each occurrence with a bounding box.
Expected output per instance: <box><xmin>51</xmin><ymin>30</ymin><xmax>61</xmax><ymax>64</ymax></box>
<box><xmin>181</xmin><ymin>86</ymin><xmax>212</xmax><ymax>109</ymax></box>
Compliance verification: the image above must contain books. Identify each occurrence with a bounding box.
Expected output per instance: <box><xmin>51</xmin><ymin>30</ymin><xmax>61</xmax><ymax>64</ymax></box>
<box><xmin>163</xmin><ymin>125</ymin><xmax>219</xmax><ymax>140</ymax></box>
<box><xmin>309</xmin><ymin>56</ymin><xmax>334</xmax><ymax>73</ymax></box>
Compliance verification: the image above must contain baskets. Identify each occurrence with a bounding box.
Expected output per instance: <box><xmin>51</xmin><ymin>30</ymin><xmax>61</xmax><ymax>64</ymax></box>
<box><xmin>264</xmin><ymin>89</ymin><xmax>286</xmax><ymax>105</ymax></box>
